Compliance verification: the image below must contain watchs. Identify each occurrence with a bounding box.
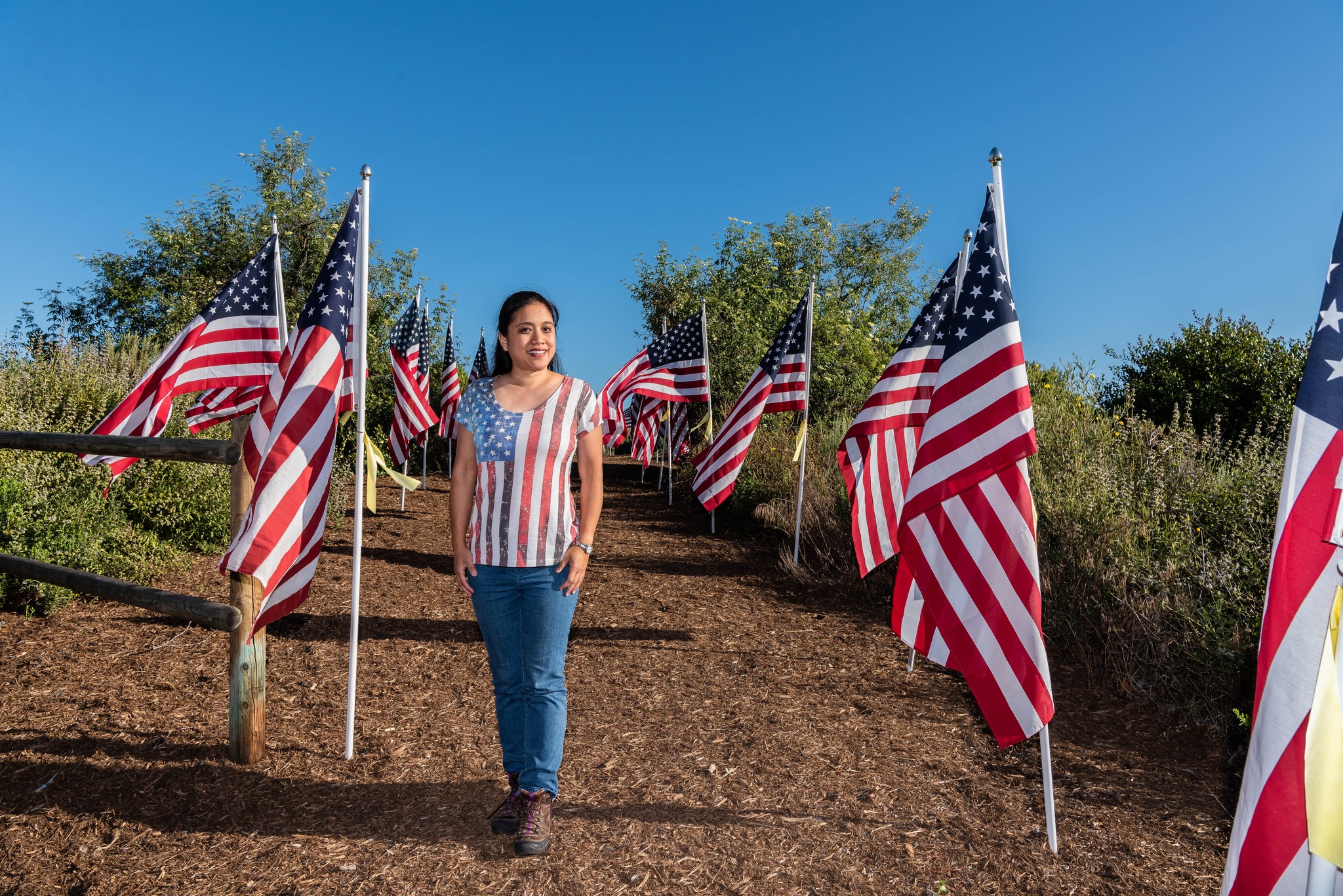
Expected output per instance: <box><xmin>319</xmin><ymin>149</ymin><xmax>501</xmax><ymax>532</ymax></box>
<box><xmin>573</xmin><ymin>540</ymin><xmax>593</xmax><ymax>554</ymax></box>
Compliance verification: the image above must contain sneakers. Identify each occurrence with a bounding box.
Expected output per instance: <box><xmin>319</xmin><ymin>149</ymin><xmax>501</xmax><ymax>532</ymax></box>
<box><xmin>485</xmin><ymin>774</ymin><xmax>522</xmax><ymax>835</ymax></box>
<box><xmin>514</xmin><ymin>787</ymin><xmax>552</xmax><ymax>857</ymax></box>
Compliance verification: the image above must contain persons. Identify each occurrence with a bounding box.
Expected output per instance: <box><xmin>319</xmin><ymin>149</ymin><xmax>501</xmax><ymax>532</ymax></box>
<box><xmin>449</xmin><ymin>291</ymin><xmax>603</xmax><ymax>856</ymax></box>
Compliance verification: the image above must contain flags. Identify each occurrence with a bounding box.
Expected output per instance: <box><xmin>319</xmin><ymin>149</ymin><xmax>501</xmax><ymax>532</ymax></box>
<box><xmin>79</xmin><ymin>186</ymin><xmax>368</xmax><ymax>643</ymax></box>
<box><xmin>597</xmin><ymin>310</ymin><xmax>710</xmax><ymax>469</ymax></box>
<box><xmin>834</xmin><ymin>184</ymin><xmax>1055</xmax><ymax>749</ymax></box>
<box><xmin>1219</xmin><ymin>208</ymin><xmax>1343</xmax><ymax>896</ymax></box>
<box><xmin>689</xmin><ymin>287</ymin><xmax>812</xmax><ymax>514</ymax></box>
<box><xmin>389</xmin><ymin>294</ymin><xmax>490</xmax><ymax>467</ymax></box>
